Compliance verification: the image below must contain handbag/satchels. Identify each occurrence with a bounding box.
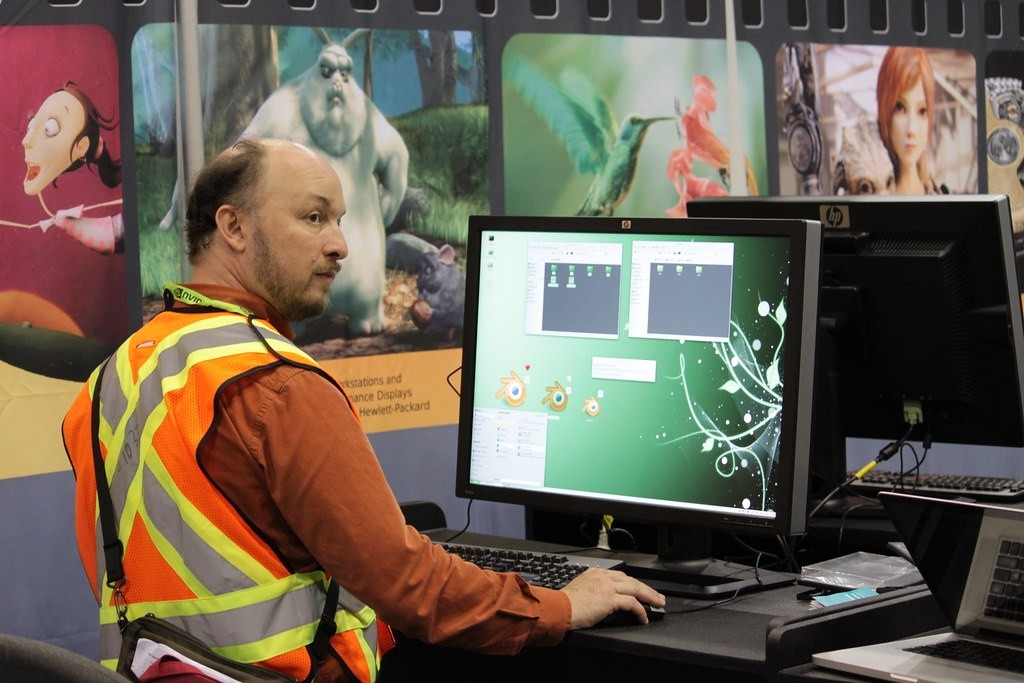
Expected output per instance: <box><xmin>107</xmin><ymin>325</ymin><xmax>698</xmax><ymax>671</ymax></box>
<box><xmin>117</xmin><ymin>613</ymin><xmax>295</xmax><ymax>683</ymax></box>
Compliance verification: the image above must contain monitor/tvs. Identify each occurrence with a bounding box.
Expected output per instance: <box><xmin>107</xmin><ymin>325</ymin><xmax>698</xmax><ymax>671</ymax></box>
<box><xmin>683</xmin><ymin>190</ymin><xmax>1023</xmax><ymax>524</ymax></box>
<box><xmin>454</xmin><ymin>215</ymin><xmax>824</xmax><ymax>597</ymax></box>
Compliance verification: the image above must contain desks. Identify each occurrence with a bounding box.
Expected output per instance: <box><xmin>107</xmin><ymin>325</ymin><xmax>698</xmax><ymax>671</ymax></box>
<box><xmin>367</xmin><ymin>499</ymin><xmax>949</xmax><ymax>683</ymax></box>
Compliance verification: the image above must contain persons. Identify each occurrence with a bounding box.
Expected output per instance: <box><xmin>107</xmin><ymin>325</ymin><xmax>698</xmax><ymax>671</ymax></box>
<box><xmin>63</xmin><ymin>136</ymin><xmax>667</xmax><ymax>683</ymax></box>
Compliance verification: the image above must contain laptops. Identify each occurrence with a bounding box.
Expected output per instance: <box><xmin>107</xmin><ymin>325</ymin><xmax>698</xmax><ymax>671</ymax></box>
<box><xmin>804</xmin><ymin>508</ymin><xmax>1023</xmax><ymax>683</ymax></box>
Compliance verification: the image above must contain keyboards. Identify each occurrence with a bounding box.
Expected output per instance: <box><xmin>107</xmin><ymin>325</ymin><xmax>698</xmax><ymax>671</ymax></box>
<box><xmin>430</xmin><ymin>539</ymin><xmax>624</xmax><ymax>592</ymax></box>
<box><xmin>842</xmin><ymin>470</ymin><xmax>1023</xmax><ymax>504</ymax></box>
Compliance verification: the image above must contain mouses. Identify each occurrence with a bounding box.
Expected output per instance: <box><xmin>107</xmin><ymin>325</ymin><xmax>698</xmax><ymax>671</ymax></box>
<box><xmin>594</xmin><ymin>598</ymin><xmax>668</xmax><ymax>630</ymax></box>
